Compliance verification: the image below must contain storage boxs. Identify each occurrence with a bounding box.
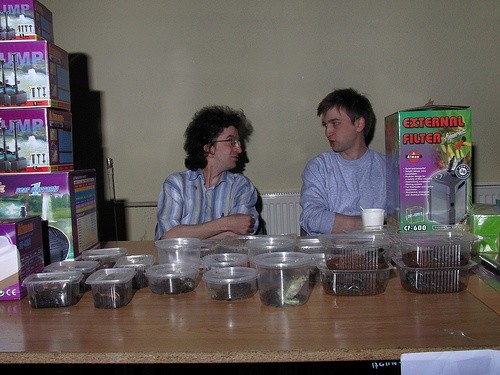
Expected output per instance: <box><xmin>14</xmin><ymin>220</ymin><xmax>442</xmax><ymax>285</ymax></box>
<box><xmin>470</xmin><ymin>203</ymin><xmax>500</xmax><ymax>252</ymax></box>
<box><xmin>0</xmin><ymin>0</ymin><xmax>155</xmax><ymax>310</ymax></box>
<box><xmin>385</xmin><ymin>100</ymin><xmax>473</xmax><ymax>235</ymax></box>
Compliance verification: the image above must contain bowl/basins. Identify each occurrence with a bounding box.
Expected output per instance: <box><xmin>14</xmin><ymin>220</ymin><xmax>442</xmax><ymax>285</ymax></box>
<box><xmin>21</xmin><ymin>228</ymin><xmax>483</xmax><ymax>309</ymax></box>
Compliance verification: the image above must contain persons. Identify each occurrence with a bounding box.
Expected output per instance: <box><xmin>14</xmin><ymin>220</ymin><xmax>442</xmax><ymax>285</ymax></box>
<box><xmin>298</xmin><ymin>89</ymin><xmax>385</xmax><ymax>234</ymax></box>
<box><xmin>155</xmin><ymin>106</ymin><xmax>259</xmax><ymax>239</ymax></box>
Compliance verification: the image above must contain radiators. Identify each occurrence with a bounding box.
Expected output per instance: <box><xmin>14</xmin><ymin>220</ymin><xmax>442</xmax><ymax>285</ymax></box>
<box><xmin>259</xmin><ymin>185</ymin><xmax>500</xmax><ymax>237</ymax></box>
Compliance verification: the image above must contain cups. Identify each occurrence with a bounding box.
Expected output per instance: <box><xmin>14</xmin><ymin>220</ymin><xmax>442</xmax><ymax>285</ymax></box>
<box><xmin>359</xmin><ymin>205</ymin><xmax>385</xmax><ymax>227</ymax></box>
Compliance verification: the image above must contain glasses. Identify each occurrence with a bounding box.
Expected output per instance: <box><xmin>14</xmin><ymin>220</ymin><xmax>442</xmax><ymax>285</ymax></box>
<box><xmin>214</xmin><ymin>136</ymin><xmax>241</xmax><ymax>147</ymax></box>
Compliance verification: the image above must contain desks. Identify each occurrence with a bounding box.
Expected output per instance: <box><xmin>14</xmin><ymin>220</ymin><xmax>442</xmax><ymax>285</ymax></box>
<box><xmin>0</xmin><ymin>241</ymin><xmax>500</xmax><ymax>375</ymax></box>
